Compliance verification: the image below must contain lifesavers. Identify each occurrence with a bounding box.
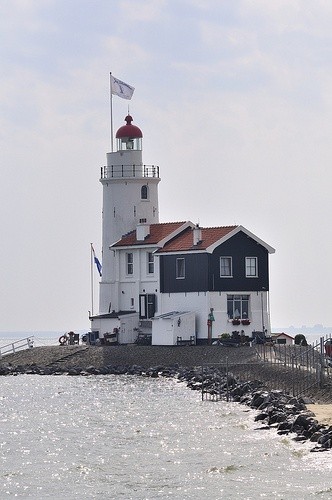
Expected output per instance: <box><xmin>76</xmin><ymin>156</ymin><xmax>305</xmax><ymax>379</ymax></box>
<box><xmin>59</xmin><ymin>336</ymin><xmax>68</xmax><ymax>343</ymax></box>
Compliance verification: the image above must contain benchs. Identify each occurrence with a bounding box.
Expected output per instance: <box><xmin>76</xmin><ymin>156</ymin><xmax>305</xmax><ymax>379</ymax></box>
<box><xmin>135</xmin><ymin>333</ymin><xmax>152</xmax><ymax>345</ymax></box>
<box><xmin>177</xmin><ymin>336</ymin><xmax>195</xmax><ymax>346</ymax></box>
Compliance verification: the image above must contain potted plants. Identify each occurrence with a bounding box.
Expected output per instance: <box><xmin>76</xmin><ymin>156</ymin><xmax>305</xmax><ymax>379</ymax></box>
<box><xmin>220</xmin><ymin>318</ymin><xmax>274</xmax><ymax>352</ymax></box>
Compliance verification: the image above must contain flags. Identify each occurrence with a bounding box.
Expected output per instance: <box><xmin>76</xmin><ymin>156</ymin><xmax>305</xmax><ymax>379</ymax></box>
<box><xmin>110</xmin><ymin>73</ymin><xmax>137</xmax><ymax>102</ymax></box>
<box><xmin>91</xmin><ymin>246</ymin><xmax>102</xmax><ymax>278</ymax></box>
